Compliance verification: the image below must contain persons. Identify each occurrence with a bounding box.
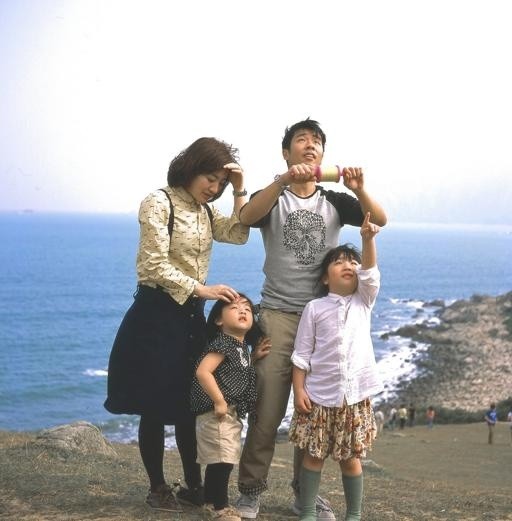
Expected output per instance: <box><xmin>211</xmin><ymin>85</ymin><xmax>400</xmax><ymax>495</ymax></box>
<box><xmin>287</xmin><ymin>210</ymin><xmax>381</xmax><ymax>521</ymax></box>
<box><xmin>375</xmin><ymin>402</ymin><xmax>415</xmax><ymax>435</ymax></box>
<box><xmin>102</xmin><ymin>136</ymin><xmax>250</xmax><ymax>514</ymax></box>
<box><xmin>508</xmin><ymin>407</ymin><xmax>512</xmax><ymax>431</ymax></box>
<box><xmin>484</xmin><ymin>404</ymin><xmax>497</xmax><ymax>444</ymax></box>
<box><xmin>189</xmin><ymin>291</ymin><xmax>272</xmax><ymax>521</ymax></box>
<box><xmin>237</xmin><ymin>116</ymin><xmax>387</xmax><ymax>521</ymax></box>
<box><xmin>425</xmin><ymin>406</ymin><xmax>435</xmax><ymax>431</ymax></box>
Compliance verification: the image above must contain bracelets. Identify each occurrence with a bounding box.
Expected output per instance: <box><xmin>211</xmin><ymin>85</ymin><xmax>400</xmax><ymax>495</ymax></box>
<box><xmin>232</xmin><ymin>188</ymin><xmax>247</xmax><ymax>196</ymax></box>
<box><xmin>274</xmin><ymin>174</ymin><xmax>287</xmax><ymax>191</ymax></box>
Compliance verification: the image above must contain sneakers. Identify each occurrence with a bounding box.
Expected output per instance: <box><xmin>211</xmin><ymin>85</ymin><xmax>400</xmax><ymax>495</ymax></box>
<box><xmin>144</xmin><ymin>487</ymin><xmax>336</xmax><ymax>520</ymax></box>
